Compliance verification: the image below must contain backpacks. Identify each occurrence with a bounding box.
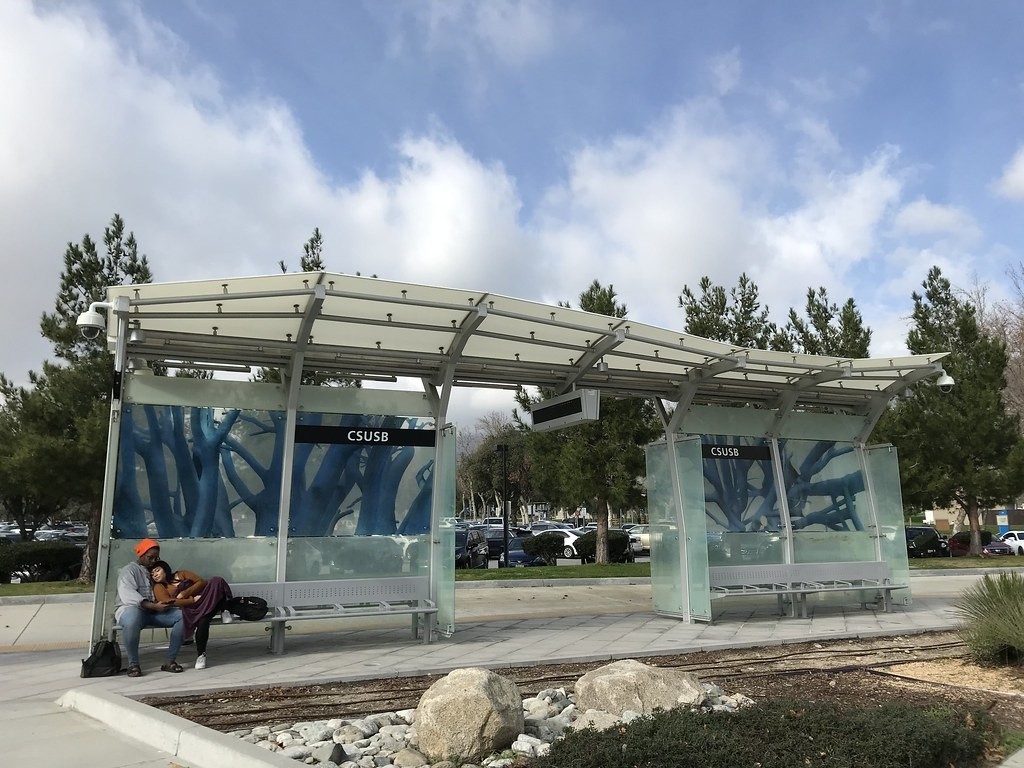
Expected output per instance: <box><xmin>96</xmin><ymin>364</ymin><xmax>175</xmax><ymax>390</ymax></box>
<box><xmin>227</xmin><ymin>596</ymin><xmax>269</xmax><ymax>621</ymax></box>
<box><xmin>81</xmin><ymin>641</ymin><xmax>122</xmax><ymax>678</ymax></box>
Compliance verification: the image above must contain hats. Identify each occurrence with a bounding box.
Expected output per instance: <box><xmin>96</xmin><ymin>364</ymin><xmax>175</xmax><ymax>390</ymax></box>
<box><xmin>134</xmin><ymin>538</ymin><xmax>160</xmax><ymax>557</ymax></box>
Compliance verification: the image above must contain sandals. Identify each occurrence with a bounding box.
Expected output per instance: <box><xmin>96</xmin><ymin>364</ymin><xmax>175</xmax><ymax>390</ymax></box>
<box><xmin>161</xmin><ymin>662</ymin><xmax>184</xmax><ymax>673</ymax></box>
<box><xmin>126</xmin><ymin>665</ymin><xmax>142</xmax><ymax>677</ymax></box>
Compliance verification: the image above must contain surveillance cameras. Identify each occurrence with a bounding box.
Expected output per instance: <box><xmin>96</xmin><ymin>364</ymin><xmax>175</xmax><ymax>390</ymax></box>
<box><xmin>76</xmin><ymin>310</ymin><xmax>105</xmax><ymax>341</ymax></box>
<box><xmin>936</xmin><ymin>375</ymin><xmax>954</xmax><ymax>393</ymax></box>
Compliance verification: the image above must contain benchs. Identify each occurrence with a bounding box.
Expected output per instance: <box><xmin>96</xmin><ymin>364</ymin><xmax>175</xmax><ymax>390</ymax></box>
<box><xmin>708</xmin><ymin>577</ymin><xmax>908</xmax><ymax>620</ymax></box>
<box><xmin>110</xmin><ymin>598</ymin><xmax>439</xmax><ymax>657</ymax></box>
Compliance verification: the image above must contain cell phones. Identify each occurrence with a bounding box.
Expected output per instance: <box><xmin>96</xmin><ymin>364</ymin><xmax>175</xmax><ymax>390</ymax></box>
<box><xmin>165</xmin><ymin>599</ymin><xmax>176</xmax><ymax>604</ymax></box>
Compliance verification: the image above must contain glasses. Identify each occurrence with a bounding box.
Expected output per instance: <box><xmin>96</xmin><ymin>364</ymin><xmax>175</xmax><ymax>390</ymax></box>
<box><xmin>143</xmin><ymin>554</ymin><xmax>160</xmax><ymax>561</ymax></box>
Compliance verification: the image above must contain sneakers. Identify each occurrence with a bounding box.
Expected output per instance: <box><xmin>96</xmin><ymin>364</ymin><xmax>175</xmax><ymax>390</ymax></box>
<box><xmin>221</xmin><ymin>610</ymin><xmax>234</xmax><ymax>623</ymax></box>
<box><xmin>195</xmin><ymin>652</ymin><xmax>207</xmax><ymax>669</ymax></box>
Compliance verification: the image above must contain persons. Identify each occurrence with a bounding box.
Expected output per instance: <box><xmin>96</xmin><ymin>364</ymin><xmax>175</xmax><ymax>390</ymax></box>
<box><xmin>150</xmin><ymin>562</ymin><xmax>235</xmax><ymax>671</ymax></box>
<box><xmin>114</xmin><ymin>538</ymin><xmax>184</xmax><ymax>677</ymax></box>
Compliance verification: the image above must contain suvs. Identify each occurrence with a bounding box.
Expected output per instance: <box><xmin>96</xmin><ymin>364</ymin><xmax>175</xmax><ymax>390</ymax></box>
<box><xmin>905</xmin><ymin>525</ymin><xmax>952</xmax><ymax>557</ymax></box>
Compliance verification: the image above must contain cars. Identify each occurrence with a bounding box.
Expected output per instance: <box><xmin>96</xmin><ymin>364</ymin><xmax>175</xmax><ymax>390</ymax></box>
<box><xmin>949</xmin><ymin>530</ymin><xmax>1012</xmax><ymax>556</ymax></box>
<box><xmin>2</xmin><ymin>514</ymin><xmax>675</xmax><ymax>569</ymax></box>
<box><xmin>999</xmin><ymin>531</ymin><xmax>1024</xmax><ymax>556</ymax></box>
